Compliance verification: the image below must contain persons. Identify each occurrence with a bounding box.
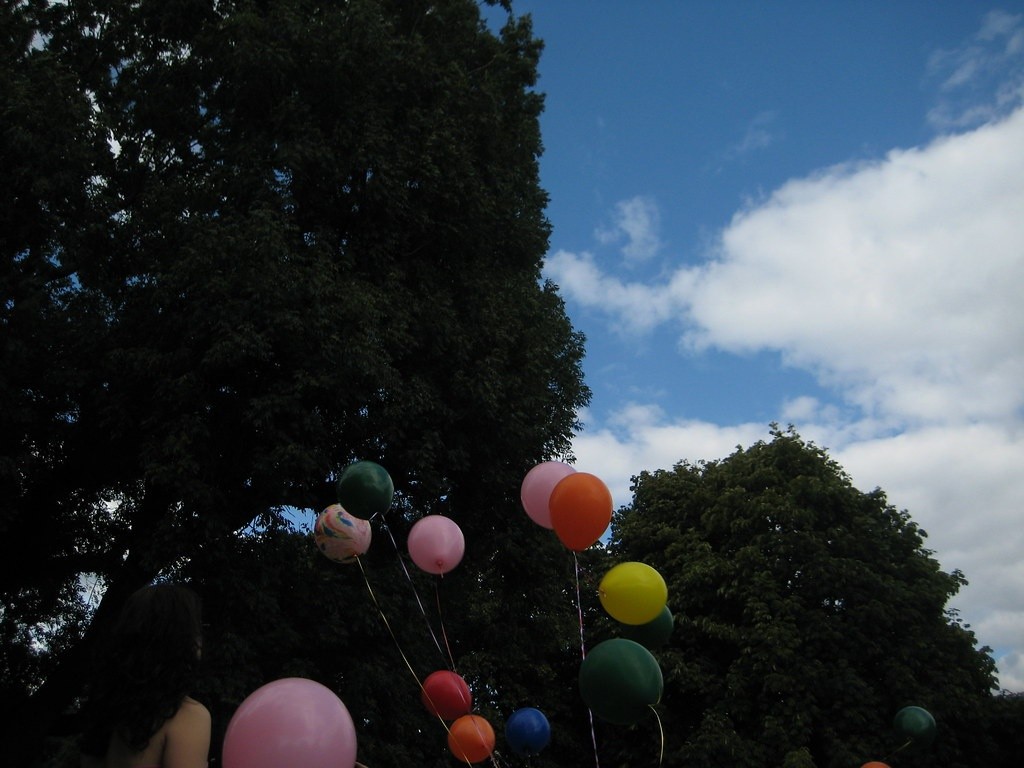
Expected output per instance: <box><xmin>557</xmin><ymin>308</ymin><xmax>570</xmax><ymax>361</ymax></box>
<box><xmin>75</xmin><ymin>584</ymin><xmax>212</xmax><ymax>768</ymax></box>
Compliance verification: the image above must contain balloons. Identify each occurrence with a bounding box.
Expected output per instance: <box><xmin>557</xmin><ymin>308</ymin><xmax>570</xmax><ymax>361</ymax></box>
<box><xmin>861</xmin><ymin>761</ymin><xmax>890</xmax><ymax>768</ymax></box>
<box><xmin>336</xmin><ymin>461</ymin><xmax>394</xmax><ymax>520</ymax></box>
<box><xmin>422</xmin><ymin>670</ymin><xmax>472</xmax><ymax>720</ymax></box>
<box><xmin>599</xmin><ymin>562</ymin><xmax>675</xmax><ymax>648</ymax></box>
<box><xmin>315</xmin><ymin>503</ymin><xmax>372</xmax><ymax>564</ymax></box>
<box><xmin>448</xmin><ymin>715</ymin><xmax>495</xmax><ymax>763</ymax></box>
<box><xmin>578</xmin><ymin>638</ymin><xmax>663</xmax><ymax>725</ymax></box>
<box><xmin>223</xmin><ymin>677</ymin><xmax>357</xmax><ymax>768</ymax></box>
<box><xmin>893</xmin><ymin>706</ymin><xmax>936</xmax><ymax>749</ymax></box>
<box><xmin>505</xmin><ymin>708</ymin><xmax>551</xmax><ymax>754</ymax></box>
<box><xmin>407</xmin><ymin>515</ymin><xmax>464</xmax><ymax>574</ymax></box>
<box><xmin>520</xmin><ymin>461</ymin><xmax>612</xmax><ymax>552</ymax></box>
